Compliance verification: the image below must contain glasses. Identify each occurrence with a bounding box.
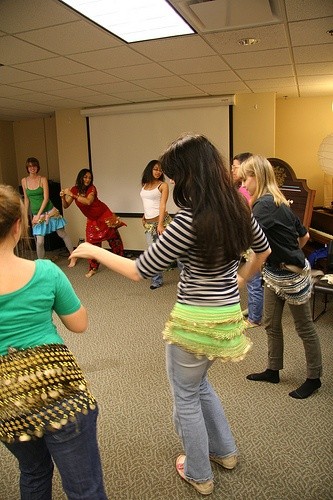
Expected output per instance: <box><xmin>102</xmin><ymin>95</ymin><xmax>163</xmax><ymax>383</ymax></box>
<box><xmin>231</xmin><ymin>165</ymin><xmax>240</xmax><ymax>171</ymax></box>
<box><xmin>153</xmin><ymin>167</ymin><xmax>163</xmax><ymax>173</ymax></box>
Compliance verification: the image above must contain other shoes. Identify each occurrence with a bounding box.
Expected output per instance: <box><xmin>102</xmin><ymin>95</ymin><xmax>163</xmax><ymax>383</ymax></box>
<box><xmin>209</xmin><ymin>454</ymin><xmax>237</xmax><ymax>468</ymax></box>
<box><xmin>177</xmin><ymin>453</ymin><xmax>214</xmax><ymax>495</ymax></box>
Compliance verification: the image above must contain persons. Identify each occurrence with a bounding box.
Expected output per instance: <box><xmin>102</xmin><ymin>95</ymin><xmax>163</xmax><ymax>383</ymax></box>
<box><xmin>228</xmin><ymin>153</ymin><xmax>269</xmax><ymax>330</ymax></box>
<box><xmin>60</xmin><ymin>169</ymin><xmax>127</xmax><ymax>277</ymax></box>
<box><xmin>140</xmin><ymin>160</ymin><xmax>181</xmax><ymax>289</ymax></box>
<box><xmin>69</xmin><ymin>135</ymin><xmax>273</xmax><ymax>495</ymax></box>
<box><xmin>18</xmin><ymin>157</ymin><xmax>78</xmax><ymax>269</ymax></box>
<box><xmin>0</xmin><ymin>182</ymin><xmax>108</xmax><ymax>500</ymax></box>
<box><xmin>236</xmin><ymin>156</ymin><xmax>324</xmax><ymax>400</ymax></box>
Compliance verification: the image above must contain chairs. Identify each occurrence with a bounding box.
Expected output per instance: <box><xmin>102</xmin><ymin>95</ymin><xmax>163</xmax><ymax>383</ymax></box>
<box><xmin>312</xmin><ymin>239</ymin><xmax>333</xmax><ymax>322</ymax></box>
